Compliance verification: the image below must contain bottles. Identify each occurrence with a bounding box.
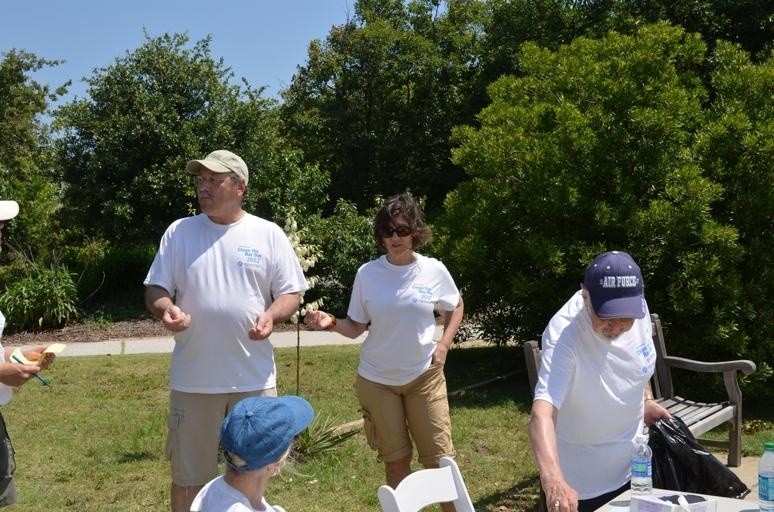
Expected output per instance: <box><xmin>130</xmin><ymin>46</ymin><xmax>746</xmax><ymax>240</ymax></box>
<box><xmin>757</xmin><ymin>441</ymin><xmax>774</xmax><ymax>512</ymax></box>
<box><xmin>631</xmin><ymin>434</ymin><xmax>653</xmax><ymax>497</ymax></box>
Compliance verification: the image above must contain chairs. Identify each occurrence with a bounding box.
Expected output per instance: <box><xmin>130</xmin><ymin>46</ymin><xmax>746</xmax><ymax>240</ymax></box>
<box><xmin>378</xmin><ymin>455</ymin><xmax>479</xmax><ymax>512</ymax></box>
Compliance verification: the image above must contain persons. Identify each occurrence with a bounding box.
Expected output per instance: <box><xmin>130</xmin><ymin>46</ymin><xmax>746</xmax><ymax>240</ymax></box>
<box><xmin>0</xmin><ymin>200</ymin><xmax>55</xmax><ymax>512</ymax></box>
<box><xmin>527</xmin><ymin>248</ymin><xmax>674</xmax><ymax>512</ymax></box>
<box><xmin>188</xmin><ymin>394</ymin><xmax>315</xmax><ymax>511</ymax></box>
<box><xmin>302</xmin><ymin>194</ymin><xmax>464</xmax><ymax>511</ymax></box>
<box><xmin>140</xmin><ymin>147</ymin><xmax>312</xmax><ymax>511</ymax></box>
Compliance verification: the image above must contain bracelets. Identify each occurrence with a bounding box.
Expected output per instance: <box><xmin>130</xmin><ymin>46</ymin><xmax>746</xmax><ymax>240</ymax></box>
<box><xmin>324</xmin><ymin>313</ymin><xmax>336</xmax><ymax>332</ymax></box>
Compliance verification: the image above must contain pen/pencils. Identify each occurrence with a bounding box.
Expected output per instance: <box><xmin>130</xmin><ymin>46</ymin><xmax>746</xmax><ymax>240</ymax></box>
<box><xmin>12</xmin><ymin>355</ymin><xmax>51</xmax><ymax>388</ymax></box>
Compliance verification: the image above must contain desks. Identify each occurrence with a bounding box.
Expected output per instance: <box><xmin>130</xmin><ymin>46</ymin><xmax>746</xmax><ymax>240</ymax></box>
<box><xmin>587</xmin><ymin>485</ymin><xmax>774</xmax><ymax>512</ymax></box>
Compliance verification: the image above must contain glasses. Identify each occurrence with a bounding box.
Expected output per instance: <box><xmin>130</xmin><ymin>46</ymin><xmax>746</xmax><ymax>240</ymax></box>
<box><xmin>196</xmin><ymin>176</ymin><xmax>224</xmax><ymax>184</ymax></box>
<box><xmin>381</xmin><ymin>226</ymin><xmax>412</xmax><ymax>238</ymax></box>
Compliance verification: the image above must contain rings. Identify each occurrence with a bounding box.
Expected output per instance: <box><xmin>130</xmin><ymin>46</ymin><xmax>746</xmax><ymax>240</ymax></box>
<box><xmin>553</xmin><ymin>503</ymin><xmax>560</xmax><ymax>508</ymax></box>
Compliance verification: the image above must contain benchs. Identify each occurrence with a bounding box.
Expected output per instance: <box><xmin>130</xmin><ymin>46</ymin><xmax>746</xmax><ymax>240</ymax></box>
<box><xmin>524</xmin><ymin>311</ymin><xmax>756</xmax><ymax>479</ymax></box>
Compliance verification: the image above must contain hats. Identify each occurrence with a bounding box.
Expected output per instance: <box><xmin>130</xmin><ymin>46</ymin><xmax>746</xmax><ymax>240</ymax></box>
<box><xmin>583</xmin><ymin>251</ymin><xmax>646</xmax><ymax>319</ymax></box>
<box><xmin>0</xmin><ymin>200</ymin><xmax>19</xmax><ymax>221</ymax></box>
<box><xmin>218</xmin><ymin>396</ymin><xmax>315</xmax><ymax>472</ymax></box>
<box><xmin>185</xmin><ymin>149</ymin><xmax>249</xmax><ymax>187</ymax></box>
